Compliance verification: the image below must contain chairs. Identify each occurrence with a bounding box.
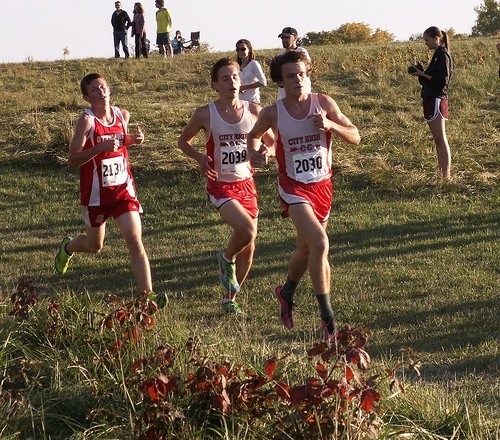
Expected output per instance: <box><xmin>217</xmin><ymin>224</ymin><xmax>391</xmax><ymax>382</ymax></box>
<box><xmin>177</xmin><ymin>31</ymin><xmax>201</xmax><ymax>54</ymax></box>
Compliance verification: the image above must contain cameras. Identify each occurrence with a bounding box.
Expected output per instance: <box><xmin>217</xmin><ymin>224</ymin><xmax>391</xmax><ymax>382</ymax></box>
<box><xmin>407</xmin><ymin>64</ymin><xmax>423</xmax><ymax>73</ymax></box>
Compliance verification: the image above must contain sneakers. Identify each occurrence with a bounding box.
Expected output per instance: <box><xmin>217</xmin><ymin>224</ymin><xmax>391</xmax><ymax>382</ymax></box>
<box><xmin>221</xmin><ymin>297</ymin><xmax>242</xmax><ymax>317</ymax></box>
<box><xmin>321</xmin><ymin>318</ymin><xmax>339</xmax><ymax>348</ymax></box>
<box><xmin>274</xmin><ymin>286</ymin><xmax>294</xmax><ymax>330</ymax></box>
<box><xmin>55</xmin><ymin>236</ymin><xmax>76</xmax><ymax>275</ymax></box>
<box><xmin>140</xmin><ymin>291</ymin><xmax>168</xmax><ymax>315</ymax></box>
<box><xmin>217</xmin><ymin>248</ymin><xmax>240</xmax><ymax>293</ymax></box>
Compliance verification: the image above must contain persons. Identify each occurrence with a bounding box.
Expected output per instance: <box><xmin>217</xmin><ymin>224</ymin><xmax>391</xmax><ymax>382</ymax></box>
<box><xmin>155</xmin><ymin>0</ymin><xmax>173</xmax><ymax>58</ymax></box>
<box><xmin>236</xmin><ymin>39</ymin><xmax>267</xmax><ymax>105</ymax></box>
<box><xmin>130</xmin><ymin>3</ymin><xmax>148</xmax><ymax>58</ymax></box>
<box><xmin>247</xmin><ymin>49</ymin><xmax>361</xmax><ymax>344</ymax></box>
<box><xmin>111</xmin><ymin>1</ymin><xmax>131</xmax><ymax>59</ymax></box>
<box><xmin>267</xmin><ymin>27</ymin><xmax>312</xmax><ymax>100</ymax></box>
<box><xmin>174</xmin><ymin>30</ymin><xmax>184</xmax><ymax>54</ymax></box>
<box><xmin>411</xmin><ymin>27</ymin><xmax>453</xmax><ymax>179</ymax></box>
<box><xmin>178</xmin><ymin>59</ymin><xmax>276</xmax><ymax>315</ymax></box>
<box><xmin>56</xmin><ymin>73</ymin><xmax>169</xmax><ymax>316</ymax></box>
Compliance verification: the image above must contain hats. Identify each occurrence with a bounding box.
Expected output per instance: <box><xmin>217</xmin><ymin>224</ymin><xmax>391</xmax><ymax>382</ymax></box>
<box><xmin>278</xmin><ymin>27</ymin><xmax>298</xmax><ymax>38</ymax></box>
